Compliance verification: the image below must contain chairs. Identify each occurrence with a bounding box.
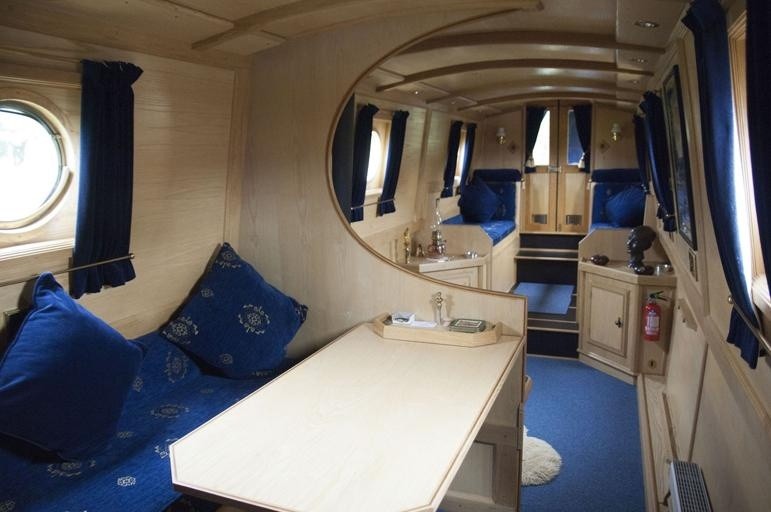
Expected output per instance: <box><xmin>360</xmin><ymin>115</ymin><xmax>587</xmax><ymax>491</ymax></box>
<box><xmin>586</xmin><ymin>169</ymin><xmax>651</xmax><ymax>235</ymax></box>
<box><xmin>441</xmin><ymin>167</ymin><xmax>522</xmax><ymax>248</ymax></box>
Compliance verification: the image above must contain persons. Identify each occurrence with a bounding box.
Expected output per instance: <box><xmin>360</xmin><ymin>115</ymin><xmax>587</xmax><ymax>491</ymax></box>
<box><xmin>626</xmin><ymin>225</ymin><xmax>656</xmax><ymax>268</ymax></box>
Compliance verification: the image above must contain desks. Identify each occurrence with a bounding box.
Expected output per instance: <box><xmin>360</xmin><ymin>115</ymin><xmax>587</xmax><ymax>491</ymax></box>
<box><xmin>167</xmin><ymin>317</ymin><xmax>527</xmax><ymax>512</ymax></box>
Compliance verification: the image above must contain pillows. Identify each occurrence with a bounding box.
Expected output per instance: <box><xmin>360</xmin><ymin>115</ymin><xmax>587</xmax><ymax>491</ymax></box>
<box><xmin>602</xmin><ymin>184</ymin><xmax>647</xmax><ymax>227</ymax></box>
<box><xmin>162</xmin><ymin>242</ymin><xmax>310</xmax><ymax>380</ymax></box>
<box><xmin>457</xmin><ymin>175</ymin><xmax>505</xmax><ymax>223</ymax></box>
<box><xmin>6</xmin><ymin>270</ymin><xmax>146</xmax><ymax>465</ymax></box>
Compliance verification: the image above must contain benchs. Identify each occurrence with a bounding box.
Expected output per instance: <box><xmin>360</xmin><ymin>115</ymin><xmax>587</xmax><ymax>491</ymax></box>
<box><xmin>0</xmin><ymin>326</ymin><xmax>285</xmax><ymax>510</ymax></box>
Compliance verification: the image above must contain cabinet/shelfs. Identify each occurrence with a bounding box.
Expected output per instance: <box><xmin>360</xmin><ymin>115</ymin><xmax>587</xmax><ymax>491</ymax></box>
<box><xmin>578</xmin><ymin>272</ymin><xmax>674</xmax><ymax>379</ymax></box>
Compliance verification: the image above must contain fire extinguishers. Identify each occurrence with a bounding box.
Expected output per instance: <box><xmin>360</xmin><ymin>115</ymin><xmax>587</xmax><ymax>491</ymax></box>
<box><xmin>641</xmin><ymin>291</ymin><xmax>667</xmax><ymax>342</ymax></box>
